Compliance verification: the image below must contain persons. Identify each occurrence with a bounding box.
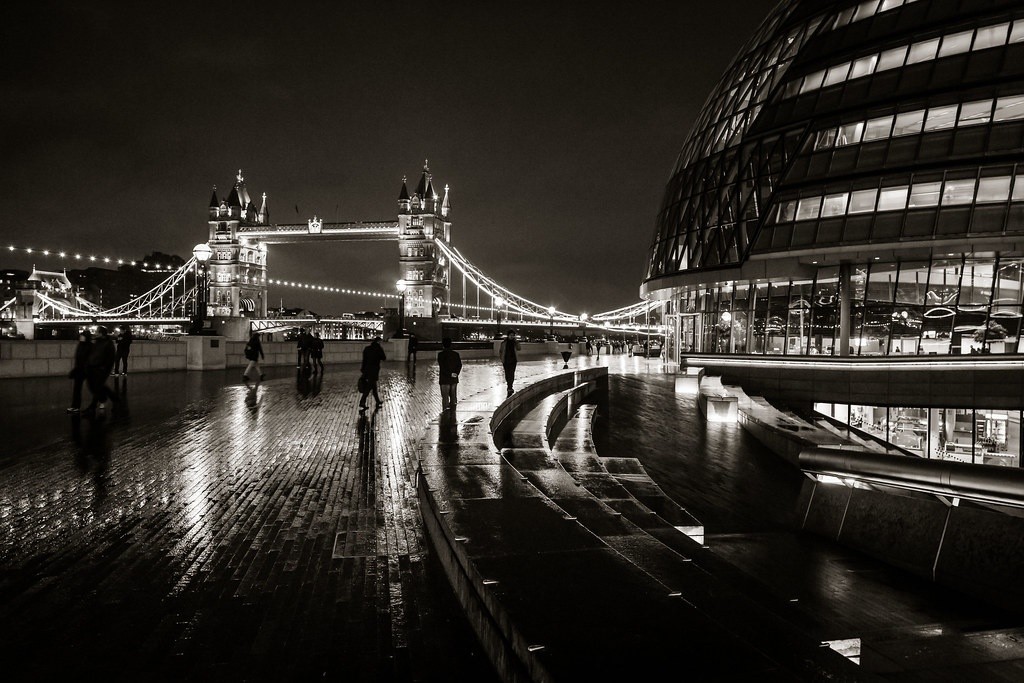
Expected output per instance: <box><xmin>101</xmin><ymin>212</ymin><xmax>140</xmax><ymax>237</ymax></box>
<box><xmin>67</xmin><ymin>326</ymin><xmax>116</xmax><ymax>411</ymax></box>
<box><xmin>896</xmin><ymin>347</ymin><xmax>900</xmax><ymax>352</ymax></box>
<box><xmin>405</xmin><ymin>333</ymin><xmax>418</xmax><ymax>364</ymax></box>
<box><xmin>242</xmin><ymin>333</ymin><xmax>266</xmax><ymax>380</ymax></box>
<box><xmin>499</xmin><ymin>329</ymin><xmax>521</xmax><ymax>390</ymax></box>
<box><xmin>971</xmin><ymin>345</ymin><xmax>975</xmax><ymax>354</ymax></box>
<box><xmin>110</xmin><ymin>325</ymin><xmax>131</xmax><ymax>376</ymax></box>
<box><xmin>296</xmin><ymin>328</ymin><xmax>324</xmax><ymax>373</ymax></box>
<box><xmin>644</xmin><ymin>342</ymin><xmax>648</xmax><ymax>355</ymax></box>
<box><xmin>437</xmin><ymin>337</ymin><xmax>462</xmax><ymax>410</ymax></box>
<box><xmin>358</xmin><ymin>337</ymin><xmax>386</xmax><ymax>408</ymax></box>
<box><xmin>628</xmin><ymin>342</ymin><xmax>633</xmax><ymax>353</ymax></box>
<box><xmin>586</xmin><ymin>340</ymin><xmax>592</xmax><ymax>356</ymax></box>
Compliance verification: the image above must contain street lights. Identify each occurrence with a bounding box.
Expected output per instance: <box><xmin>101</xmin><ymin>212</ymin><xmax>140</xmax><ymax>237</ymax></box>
<box><xmin>580</xmin><ymin>313</ymin><xmax>587</xmax><ymax>342</ymax></box>
<box><xmin>547</xmin><ymin>306</ymin><xmax>556</xmax><ymax>341</ymax></box>
<box><xmin>493</xmin><ymin>297</ymin><xmax>505</xmax><ymax>340</ymax></box>
<box><xmin>621</xmin><ymin>324</ymin><xmax>627</xmax><ymax>345</ymax></box>
<box><xmin>187</xmin><ymin>243</ymin><xmax>217</xmax><ymax>336</ymax></box>
<box><xmin>391</xmin><ymin>279</ymin><xmax>416</xmax><ymax>339</ymax></box>
<box><xmin>634</xmin><ymin>326</ymin><xmax>640</xmax><ymax>345</ymax></box>
<box><xmin>604</xmin><ymin>322</ymin><xmax>610</xmax><ymax>345</ymax></box>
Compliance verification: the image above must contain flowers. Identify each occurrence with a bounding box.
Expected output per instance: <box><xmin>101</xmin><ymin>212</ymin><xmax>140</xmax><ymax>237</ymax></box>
<box><xmin>971</xmin><ymin>319</ymin><xmax>1007</xmax><ymax>342</ymax></box>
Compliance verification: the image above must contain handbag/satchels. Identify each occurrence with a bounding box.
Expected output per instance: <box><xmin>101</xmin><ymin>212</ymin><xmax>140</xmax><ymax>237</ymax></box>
<box><xmin>358</xmin><ymin>375</ymin><xmax>368</xmax><ymax>394</ymax></box>
<box><xmin>67</xmin><ymin>368</ymin><xmax>75</xmax><ymax>380</ymax></box>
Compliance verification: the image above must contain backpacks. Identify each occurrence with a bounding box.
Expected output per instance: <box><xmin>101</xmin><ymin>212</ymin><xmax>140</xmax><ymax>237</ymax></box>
<box><xmin>244</xmin><ymin>342</ymin><xmax>253</xmax><ymax>359</ymax></box>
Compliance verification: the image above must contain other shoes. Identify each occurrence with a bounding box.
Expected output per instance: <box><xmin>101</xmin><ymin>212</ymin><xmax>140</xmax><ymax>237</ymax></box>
<box><xmin>313</xmin><ymin>370</ymin><xmax>317</xmax><ymax>373</ymax></box>
<box><xmin>360</xmin><ymin>403</ymin><xmax>369</xmax><ymax>409</ymax></box>
<box><xmin>321</xmin><ymin>367</ymin><xmax>325</xmax><ymax>371</ymax></box>
<box><xmin>243</xmin><ymin>375</ymin><xmax>250</xmax><ymax>380</ymax></box>
<box><xmin>406</xmin><ymin>361</ymin><xmax>410</xmax><ymax>364</ymax></box>
<box><xmin>66</xmin><ymin>407</ymin><xmax>78</xmax><ymax>412</ymax></box>
<box><xmin>260</xmin><ymin>374</ymin><xmax>265</xmax><ymax>379</ymax></box>
<box><xmin>308</xmin><ymin>366</ymin><xmax>311</xmax><ymax>368</ymax></box>
<box><xmin>413</xmin><ymin>363</ymin><xmax>416</xmax><ymax>366</ymax></box>
<box><xmin>99</xmin><ymin>403</ymin><xmax>105</xmax><ymax>408</ymax></box>
<box><xmin>110</xmin><ymin>372</ymin><xmax>119</xmax><ymax>377</ymax></box>
<box><xmin>376</xmin><ymin>400</ymin><xmax>383</xmax><ymax>406</ymax></box>
<box><xmin>303</xmin><ymin>365</ymin><xmax>306</xmax><ymax>368</ymax></box>
<box><xmin>297</xmin><ymin>365</ymin><xmax>300</xmax><ymax>368</ymax></box>
<box><xmin>121</xmin><ymin>373</ymin><xmax>128</xmax><ymax>377</ymax></box>
